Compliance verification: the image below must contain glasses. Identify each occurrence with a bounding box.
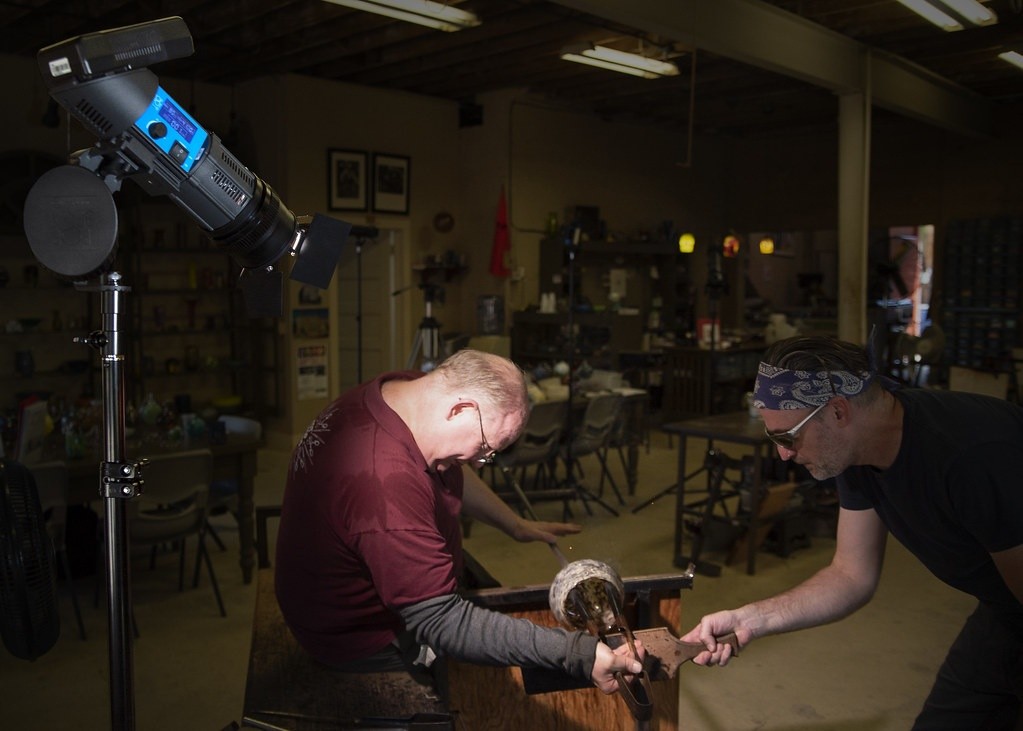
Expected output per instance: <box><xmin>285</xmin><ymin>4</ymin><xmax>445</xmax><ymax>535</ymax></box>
<box><xmin>457</xmin><ymin>397</ymin><xmax>494</xmax><ymax>465</ymax></box>
<box><xmin>764</xmin><ymin>402</ymin><xmax>829</xmax><ymax>450</ymax></box>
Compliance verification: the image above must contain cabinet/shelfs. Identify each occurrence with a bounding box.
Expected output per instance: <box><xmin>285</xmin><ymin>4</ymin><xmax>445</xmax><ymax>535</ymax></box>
<box><xmin>0</xmin><ymin>147</ymin><xmax>280</xmax><ymax>419</ymax></box>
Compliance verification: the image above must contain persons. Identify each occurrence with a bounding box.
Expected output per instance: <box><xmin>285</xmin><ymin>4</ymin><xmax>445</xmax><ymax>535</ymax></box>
<box><xmin>274</xmin><ymin>348</ymin><xmax>645</xmax><ymax>694</ymax></box>
<box><xmin>680</xmin><ymin>334</ymin><xmax>1023</xmax><ymax>731</ymax></box>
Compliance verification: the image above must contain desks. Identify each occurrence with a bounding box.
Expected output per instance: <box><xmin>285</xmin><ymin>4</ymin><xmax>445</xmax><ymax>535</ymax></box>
<box><xmin>17</xmin><ymin>416</ymin><xmax>262</xmax><ymax>586</ymax></box>
<box><xmin>460</xmin><ymin>387</ymin><xmax>647</xmax><ymax>540</ymax></box>
<box><xmin>619</xmin><ymin>340</ymin><xmax>770</xmax><ymax>418</ymax></box>
<box><xmin>660</xmin><ymin>409</ymin><xmax>839</xmax><ymax>578</ymax></box>
<box><xmin>242</xmin><ymin>574</ymin><xmax>695</xmax><ymax>731</ymax></box>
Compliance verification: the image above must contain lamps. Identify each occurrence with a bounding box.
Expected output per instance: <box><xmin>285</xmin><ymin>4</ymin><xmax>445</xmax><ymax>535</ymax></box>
<box><xmin>997</xmin><ymin>51</ymin><xmax>1023</xmax><ymax>69</ymax></box>
<box><xmin>896</xmin><ymin>0</ymin><xmax>965</xmax><ymax>32</ymax></box>
<box><xmin>558</xmin><ymin>43</ymin><xmax>663</xmax><ymax>81</ymax></box>
<box><xmin>320</xmin><ymin>0</ymin><xmax>463</xmax><ymax>32</ymax></box>
<box><xmin>368</xmin><ymin>0</ymin><xmax>484</xmax><ymax>29</ymax></box>
<box><xmin>939</xmin><ymin>0</ymin><xmax>998</xmax><ymax>27</ymax></box>
<box><xmin>577</xmin><ymin>28</ymin><xmax>687</xmax><ymax>77</ymax></box>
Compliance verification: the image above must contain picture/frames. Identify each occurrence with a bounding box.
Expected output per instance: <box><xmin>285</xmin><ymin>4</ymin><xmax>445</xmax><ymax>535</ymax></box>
<box><xmin>372</xmin><ymin>152</ymin><xmax>411</xmax><ymax>215</ymax></box>
<box><xmin>327</xmin><ymin>148</ymin><xmax>369</xmax><ymax>212</ymax></box>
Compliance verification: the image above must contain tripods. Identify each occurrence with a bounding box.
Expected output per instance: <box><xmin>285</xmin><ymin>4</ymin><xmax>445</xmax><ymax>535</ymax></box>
<box><xmin>392</xmin><ymin>278</ymin><xmax>449</xmax><ymax>371</ymax></box>
<box><xmin>632</xmin><ymin>246</ymin><xmax>738</xmax><ymax>517</ymax></box>
<box><xmin>550</xmin><ymin>243</ymin><xmax>618</xmax><ymax>523</ymax></box>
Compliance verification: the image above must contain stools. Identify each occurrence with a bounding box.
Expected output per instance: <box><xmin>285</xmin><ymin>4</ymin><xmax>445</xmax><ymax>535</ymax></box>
<box><xmin>255</xmin><ymin>480</ymin><xmax>283</xmax><ymax>569</ymax></box>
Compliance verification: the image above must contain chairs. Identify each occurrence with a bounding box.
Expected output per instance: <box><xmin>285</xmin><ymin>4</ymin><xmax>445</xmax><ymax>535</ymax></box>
<box><xmin>567</xmin><ymin>369</ymin><xmax>634</xmax><ymax>501</ymax></box>
<box><xmin>172</xmin><ymin>417</ymin><xmax>261</xmax><ymax>591</ymax></box>
<box><xmin>27</xmin><ymin>459</ymin><xmax>107</xmax><ymax>643</ymax></box>
<box><xmin>127</xmin><ymin>449</ymin><xmax>229</xmax><ymax>619</ymax></box>
<box><xmin>533</xmin><ymin>392</ymin><xmax>627</xmax><ymax>520</ymax></box>
<box><xmin>478</xmin><ymin>397</ymin><xmax>596</xmax><ymax>522</ymax></box>
